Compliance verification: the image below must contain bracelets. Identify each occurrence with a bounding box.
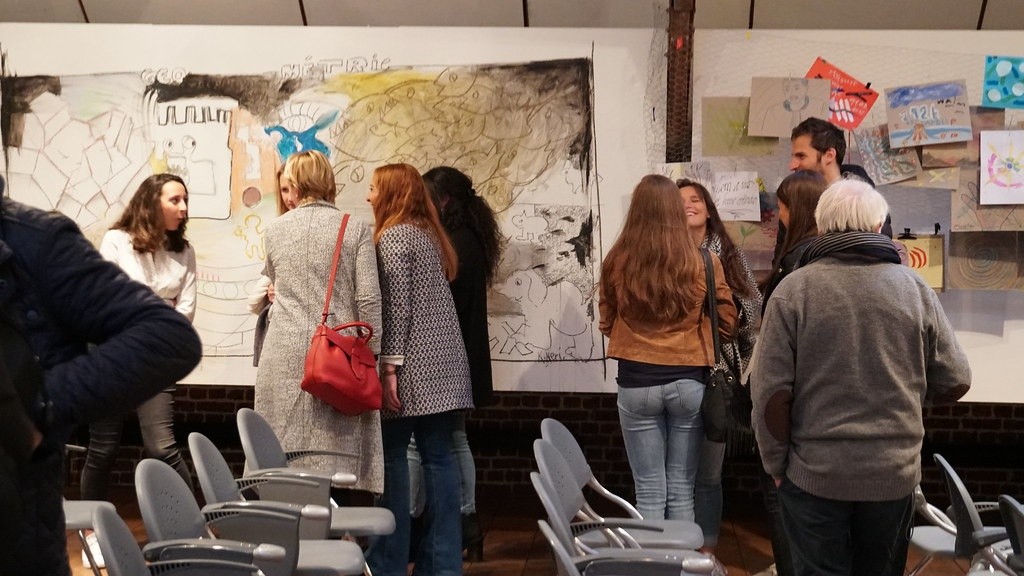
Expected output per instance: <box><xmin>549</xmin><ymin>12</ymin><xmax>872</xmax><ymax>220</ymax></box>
<box><xmin>383</xmin><ymin>370</ymin><xmax>396</xmax><ymax>375</ymax></box>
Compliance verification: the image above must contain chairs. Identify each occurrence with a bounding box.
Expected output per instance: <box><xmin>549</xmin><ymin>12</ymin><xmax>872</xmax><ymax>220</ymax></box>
<box><xmin>910</xmin><ymin>453</ymin><xmax>1024</xmax><ymax>576</ymax></box>
<box><xmin>530</xmin><ymin>418</ymin><xmax>728</xmax><ymax>576</ymax></box>
<box><xmin>62</xmin><ymin>407</ymin><xmax>395</xmax><ymax>576</ymax></box>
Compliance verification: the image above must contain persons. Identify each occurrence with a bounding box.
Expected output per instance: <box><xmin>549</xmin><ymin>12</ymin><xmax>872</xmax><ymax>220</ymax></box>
<box><xmin>762</xmin><ymin>117</ymin><xmax>892</xmax><ymax>317</ymax></box>
<box><xmin>750</xmin><ymin>166</ymin><xmax>972</xmax><ymax>576</ymax></box>
<box><xmin>599</xmin><ymin>173</ymin><xmax>742</xmax><ymax>550</ymax></box>
<box><xmin>249</xmin><ymin>148</ymin><xmax>385</xmax><ymax>540</ymax></box>
<box><xmin>367</xmin><ymin>163</ymin><xmax>476</xmax><ymax>576</ymax></box>
<box><xmin>74</xmin><ymin>174</ymin><xmax>196</xmax><ymax>573</ymax></box>
<box><xmin>1</xmin><ymin>177</ymin><xmax>203</xmax><ymax>576</ymax></box>
<box><xmin>678</xmin><ymin>180</ymin><xmax>764</xmax><ymax>576</ymax></box>
<box><xmin>419</xmin><ymin>165</ymin><xmax>506</xmax><ymax>565</ymax></box>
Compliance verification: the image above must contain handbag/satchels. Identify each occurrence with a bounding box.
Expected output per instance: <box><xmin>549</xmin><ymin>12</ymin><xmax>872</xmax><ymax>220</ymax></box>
<box><xmin>300</xmin><ymin>321</ymin><xmax>383</xmax><ymax>417</ymax></box>
<box><xmin>699</xmin><ymin>368</ymin><xmax>745</xmax><ymax>442</ymax></box>
